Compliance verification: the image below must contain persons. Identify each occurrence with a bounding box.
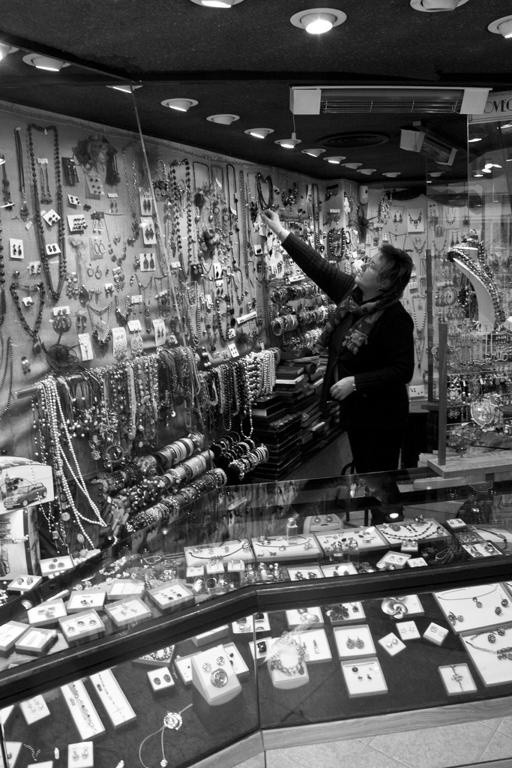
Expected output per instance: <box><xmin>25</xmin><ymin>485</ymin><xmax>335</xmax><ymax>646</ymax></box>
<box><xmin>260</xmin><ymin>206</ymin><xmax>418</xmax><ymax>527</ymax></box>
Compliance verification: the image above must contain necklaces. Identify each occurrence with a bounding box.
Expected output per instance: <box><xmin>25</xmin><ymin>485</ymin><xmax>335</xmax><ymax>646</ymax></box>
<box><xmin>0</xmin><ymin>121</ymin><xmax>512</xmax><ymax>768</ymax></box>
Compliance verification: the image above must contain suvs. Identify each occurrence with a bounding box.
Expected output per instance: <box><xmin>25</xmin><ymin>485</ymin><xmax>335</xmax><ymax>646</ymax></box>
<box><xmin>3</xmin><ymin>482</ymin><xmax>47</xmax><ymax>510</ymax></box>
